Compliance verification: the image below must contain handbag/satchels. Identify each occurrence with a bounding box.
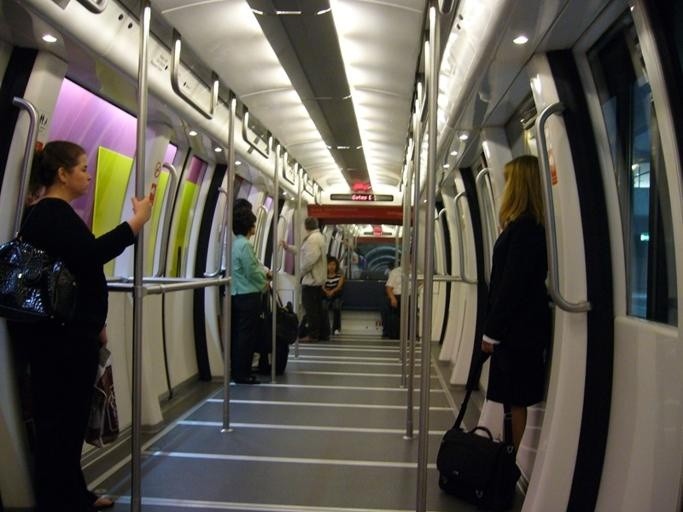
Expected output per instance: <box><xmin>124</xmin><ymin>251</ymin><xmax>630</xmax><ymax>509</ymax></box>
<box><xmin>437</xmin><ymin>428</ymin><xmax>520</xmax><ymax>508</ymax></box>
<box><xmin>0</xmin><ymin>240</ymin><xmax>76</xmax><ymax>328</ymax></box>
<box><xmin>259</xmin><ymin>311</ymin><xmax>288</xmax><ymax>375</ymax></box>
<box><xmin>381</xmin><ymin>314</ymin><xmax>409</xmax><ymax>339</ymax></box>
<box><xmin>299</xmin><ymin>312</ymin><xmax>330</xmax><ymax>341</ymax></box>
<box><xmin>255</xmin><ymin>309</ymin><xmax>298</xmax><ymax>344</ymax></box>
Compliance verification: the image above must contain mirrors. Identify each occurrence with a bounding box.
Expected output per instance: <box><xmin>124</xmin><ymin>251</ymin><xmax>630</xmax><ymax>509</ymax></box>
<box><xmin>320</xmin><ymin>222</ymin><xmax>413</xmax><ymax>280</ymax></box>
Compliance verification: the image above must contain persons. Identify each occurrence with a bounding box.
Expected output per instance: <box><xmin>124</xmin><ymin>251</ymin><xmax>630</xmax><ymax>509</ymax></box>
<box><xmin>231</xmin><ymin>207</ymin><xmax>269</xmax><ymax>384</ymax></box>
<box><xmin>14</xmin><ymin>139</ymin><xmax>156</xmax><ymax>508</ymax></box>
<box><xmin>321</xmin><ymin>256</ymin><xmax>346</xmax><ymax>336</ymax></box>
<box><xmin>280</xmin><ymin>215</ymin><xmax>329</xmax><ymax>343</ymax></box>
<box><xmin>234</xmin><ymin>198</ymin><xmax>273</xmax><ymax>282</ymax></box>
<box><xmin>382</xmin><ymin>254</ymin><xmax>422</xmax><ymax>339</ymax></box>
<box><xmin>478</xmin><ymin>152</ymin><xmax>556</xmax><ymax>452</ymax></box>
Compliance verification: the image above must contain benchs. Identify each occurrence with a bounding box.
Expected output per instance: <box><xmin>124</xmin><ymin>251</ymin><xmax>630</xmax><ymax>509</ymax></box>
<box><xmin>329</xmin><ymin>282</ymin><xmax>388</xmax><ymax>311</ymax></box>
